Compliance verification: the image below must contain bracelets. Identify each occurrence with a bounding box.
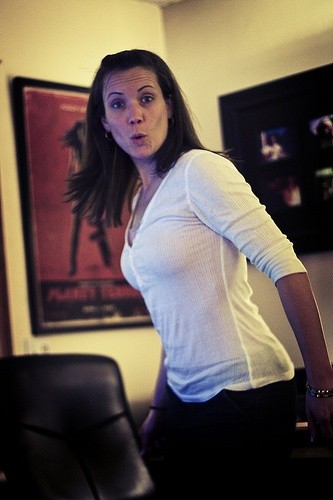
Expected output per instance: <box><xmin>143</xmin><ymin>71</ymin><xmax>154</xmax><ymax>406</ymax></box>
<box><xmin>149</xmin><ymin>405</ymin><xmax>162</xmax><ymax>412</ymax></box>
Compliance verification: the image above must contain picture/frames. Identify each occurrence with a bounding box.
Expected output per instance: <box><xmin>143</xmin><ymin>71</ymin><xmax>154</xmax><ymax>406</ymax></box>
<box><xmin>12</xmin><ymin>76</ymin><xmax>156</xmax><ymax>334</ymax></box>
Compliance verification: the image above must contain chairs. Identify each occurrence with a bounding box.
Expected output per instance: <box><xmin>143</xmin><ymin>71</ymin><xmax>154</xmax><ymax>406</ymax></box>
<box><xmin>0</xmin><ymin>353</ymin><xmax>156</xmax><ymax>500</ymax></box>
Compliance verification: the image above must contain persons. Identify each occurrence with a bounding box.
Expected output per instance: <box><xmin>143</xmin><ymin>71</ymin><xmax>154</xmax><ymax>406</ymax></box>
<box><xmin>62</xmin><ymin>119</ymin><xmax>114</xmax><ymax>275</ymax></box>
<box><xmin>65</xmin><ymin>48</ymin><xmax>333</xmax><ymax>500</ymax></box>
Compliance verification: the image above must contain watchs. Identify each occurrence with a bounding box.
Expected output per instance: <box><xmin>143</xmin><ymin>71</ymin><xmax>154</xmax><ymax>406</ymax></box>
<box><xmin>306</xmin><ymin>379</ymin><xmax>333</xmax><ymax>400</ymax></box>
